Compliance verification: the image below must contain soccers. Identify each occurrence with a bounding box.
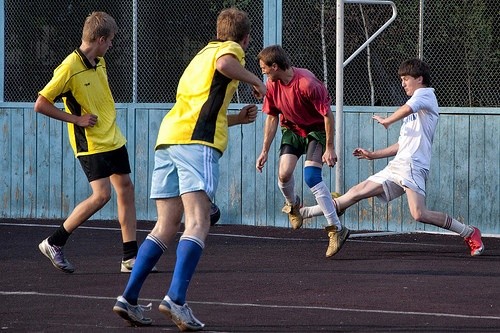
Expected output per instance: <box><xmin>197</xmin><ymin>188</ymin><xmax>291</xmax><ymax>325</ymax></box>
<box><xmin>209</xmin><ymin>204</ymin><xmax>221</xmax><ymax>227</ymax></box>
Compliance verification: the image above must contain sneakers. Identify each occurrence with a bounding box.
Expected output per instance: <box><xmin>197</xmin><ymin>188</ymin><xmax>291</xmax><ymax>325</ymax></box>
<box><xmin>325</xmin><ymin>225</ymin><xmax>351</xmax><ymax>257</ymax></box>
<box><xmin>37</xmin><ymin>236</ymin><xmax>74</xmax><ymax>273</ymax></box>
<box><xmin>463</xmin><ymin>225</ymin><xmax>484</xmax><ymax>257</ymax></box>
<box><xmin>121</xmin><ymin>256</ymin><xmax>158</xmax><ymax>273</ymax></box>
<box><xmin>112</xmin><ymin>296</ymin><xmax>153</xmax><ymax>326</ymax></box>
<box><xmin>158</xmin><ymin>294</ymin><xmax>205</xmax><ymax>332</ymax></box>
<box><xmin>282</xmin><ymin>195</ymin><xmax>304</xmax><ymax>231</ymax></box>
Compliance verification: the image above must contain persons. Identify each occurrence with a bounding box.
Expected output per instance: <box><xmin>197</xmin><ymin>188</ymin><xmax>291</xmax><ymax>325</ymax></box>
<box><xmin>33</xmin><ymin>11</ymin><xmax>140</xmax><ymax>273</ymax></box>
<box><xmin>300</xmin><ymin>59</ymin><xmax>485</xmax><ymax>256</ymax></box>
<box><xmin>113</xmin><ymin>8</ymin><xmax>267</xmax><ymax>330</ymax></box>
<box><xmin>256</xmin><ymin>44</ymin><xmax>351</xmax><ymax>257</ymax></box>
